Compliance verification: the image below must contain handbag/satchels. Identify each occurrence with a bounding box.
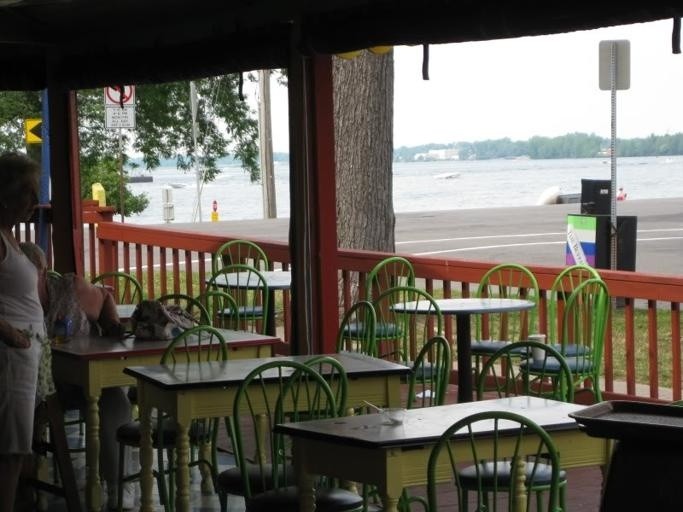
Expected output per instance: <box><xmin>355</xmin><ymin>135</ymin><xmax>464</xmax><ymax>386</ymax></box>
<box><xmin>132</xmin><ymin>300</ymin><xmax>194</xmax><ymax>340</ymax></box>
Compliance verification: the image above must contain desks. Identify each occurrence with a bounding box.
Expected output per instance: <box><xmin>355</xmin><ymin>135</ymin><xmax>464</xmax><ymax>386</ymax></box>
<box><xmin>124</xmin><ymin>348</ymin><xmax>411</xmax><ymax>512</ymax></box>
<box><xmin>275</xmin><ymin>396</ymin><xmax>612</xmax><ymax>511</ymax></box>
<box><xmin>48</xmin><ymin>327</ymin><xmax>284</xmax><ymax>512</ymax></box>
<box><xmin>203</xmin><ymin>270</ymin><xmax>291</xmax><ymax>340</ymax></box>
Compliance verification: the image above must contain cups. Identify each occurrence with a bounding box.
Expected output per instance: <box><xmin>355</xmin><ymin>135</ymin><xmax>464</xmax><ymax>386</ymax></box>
<box><xmin>528</xmin><ymin>333</ymin><xmax>546</xmax><ymax>363</ymax></box>
<box><xmin>54</xmin><ymin>320</ymin><xmax>71</xmax><ymax>344</ymax></box>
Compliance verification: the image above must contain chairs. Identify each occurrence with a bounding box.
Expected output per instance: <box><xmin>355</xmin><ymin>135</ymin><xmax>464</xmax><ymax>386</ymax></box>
<box><xmin>118</xmin><ymin>325</ymin><xmax>228</xmax><ymax>512</ymax></box>
<box><xmin>233</xmin><ymin>361</ymin><xmax>365</xmax><ymax>512</ymax></box>
<box><xmin>86</xmin><ymin>240</ymin><xmax>270</xmax><ymax>339</ymax></box>
<box><xmin>428</xmin><ymin>410</ymin><xmax>566</xmax><ymax>511</ymax></box>
<box><xmin>337</xmin><ymin>257</ymin><xmax>610</xmax><ymax>408</ymax></box>
<box><xmin>458</xmin><ymin>343</ymin><xmax>574</xmax><ymax>511</ymax></box>
<box><xmin>216</xmin><ymin>354</ymin><xmax>349</xmax><ymax>511</ymax></box>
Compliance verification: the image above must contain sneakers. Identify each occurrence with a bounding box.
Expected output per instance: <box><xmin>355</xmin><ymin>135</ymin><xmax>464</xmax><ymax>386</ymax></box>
<box><xmin>111</xmin><ymin>480</ymin><xmax>135</xmax><ymax>509</ymax></box>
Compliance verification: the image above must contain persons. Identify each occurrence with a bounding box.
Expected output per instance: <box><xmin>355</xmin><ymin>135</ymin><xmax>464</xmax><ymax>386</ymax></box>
<box><xmin>616</xmin><ymin>187</ymin><xmax>626</xmax><ymax>200</ymax></box>
<box><xmin>18</xmin><ymin>238</ymin><xmax>132</xmax><ymax>512</ymax></box>
<box><xmin>0</xmin><ymin>151</ymin><xmax>45</xmax><ymax>512</ymax></box>
